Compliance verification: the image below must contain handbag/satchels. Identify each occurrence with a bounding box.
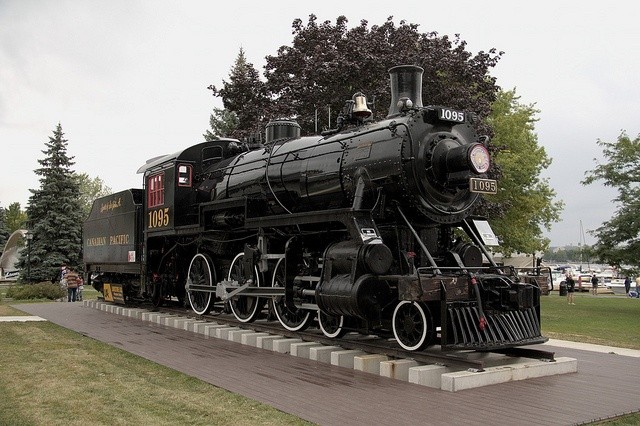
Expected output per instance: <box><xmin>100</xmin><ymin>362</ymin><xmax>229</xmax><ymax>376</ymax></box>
<box><xmin>568</xmin><ymin>285</ymin><xmax>574</xmax><ymax>292</ymax></box>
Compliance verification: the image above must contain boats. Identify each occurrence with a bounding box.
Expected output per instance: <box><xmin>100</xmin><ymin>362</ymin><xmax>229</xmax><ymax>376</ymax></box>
<box><xmin>609</xmin><ymin>268</ymin><xmax>637</xmax><ymax>295</ymax></box>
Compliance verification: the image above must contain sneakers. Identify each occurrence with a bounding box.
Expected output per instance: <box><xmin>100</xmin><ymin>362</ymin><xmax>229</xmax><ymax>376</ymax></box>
<box><xmin>72</xmin><ymin>299</ymin><xmax>75</xmax><ymax>301</ymax></box>
<box><xmin>68</xmin><ymin>300</ymin><xmax>70</xmax><ymax>302</ymax></box>
<box><xmin>568</xmin><ymin>303</ymin><xmax>570</xmax><ymax>304</ymax></box>
<box><xmin>571</xmin><ymin>303</ymin><xmax>576</xmax><ymax>305</ymax></box>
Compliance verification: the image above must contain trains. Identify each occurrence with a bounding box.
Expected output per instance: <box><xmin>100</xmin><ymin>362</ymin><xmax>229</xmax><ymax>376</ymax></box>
<box><xmin>81</xmin><ymin>62</ymin><xmax>556</xmax><ymax>370</ymax></box>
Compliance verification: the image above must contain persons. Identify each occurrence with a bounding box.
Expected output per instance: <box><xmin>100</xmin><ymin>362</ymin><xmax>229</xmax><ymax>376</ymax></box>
<box><xmin>66</xmin><ymin>268</ymin><xmax>80</xmax><ymax>303</ymax></box>
<box><xmin>566</xmin><ymin>275</ymin><xmax>576</xmax><ymax>306</ymax></box>
<box><xmin>624</xmin><ymin>276</ymin><xmax>631</xmax><ymax>296</ymax></box>
<box><xmin>591</xmin><ymin>274</ymin><xmax>599</xmax><ymax>295</ymax></box>
<box><xmin>635</xmin><ymin>274</ymin><xmax>640</xmax><ymax>297</ymax></box>
<box><xmin>79</xmin><ymin>275</ymin><xmax>84</xmax><ymax>302</ymax></box>
